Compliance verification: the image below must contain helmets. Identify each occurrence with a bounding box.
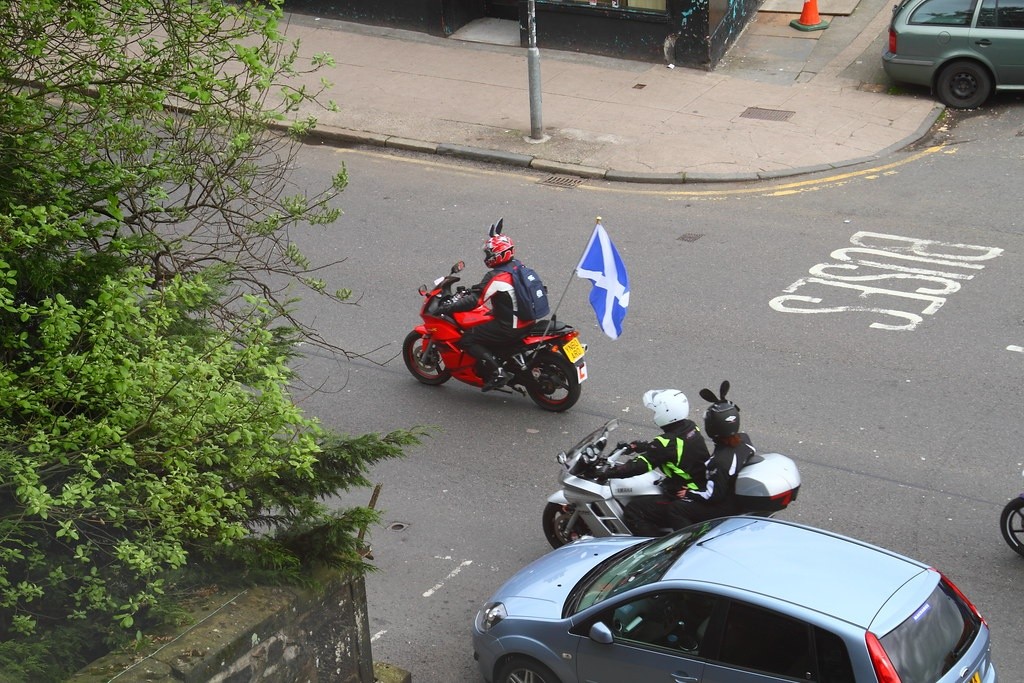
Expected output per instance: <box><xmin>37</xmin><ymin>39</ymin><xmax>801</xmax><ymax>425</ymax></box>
<box><xmin>481</xmin><ymin>216</ymin><xmax>516</xmax><ymax>269</ymax></box>
<box><xmin>700</xmin><ymin>380</ymin><xmax>741</xmax><ymax>441</ymax></box>
<box><xmin>641</xmin><ymin>387</ymin><xmax>691</xmax><ymax>428</ymax></box>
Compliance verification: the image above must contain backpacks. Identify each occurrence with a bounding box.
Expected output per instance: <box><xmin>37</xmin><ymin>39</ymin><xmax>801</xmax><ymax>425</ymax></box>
<box><xmin>496</xmin><ymin>260</ymin><xmax>551</xmax><ymax>321</ymax></box>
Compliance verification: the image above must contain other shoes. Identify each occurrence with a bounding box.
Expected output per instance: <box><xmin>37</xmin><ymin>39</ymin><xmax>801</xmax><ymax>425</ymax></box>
<box><xmin>475</xmin><ymin>351</ymin><xmax>514</xmax><ymax>392</ymax></box>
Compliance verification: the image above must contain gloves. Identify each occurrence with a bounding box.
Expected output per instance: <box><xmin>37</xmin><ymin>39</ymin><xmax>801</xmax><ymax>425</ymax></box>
<box><xmin>597</xmin><ymin>467</ymin><xmax>622</xmax><ymax>481</ymax></box>
<box><xmin>432</xmin><ymin>294</ymin><xmax>478</xmax><ymax>315</ymax></box>
<box><xmin>630</xmin><ymin>439</ymin><xmax>648</xmax><ymax>453</ymax></box>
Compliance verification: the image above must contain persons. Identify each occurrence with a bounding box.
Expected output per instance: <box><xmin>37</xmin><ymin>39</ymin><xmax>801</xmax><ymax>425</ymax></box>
<box><xmin>596</xmin><ymin>380</ymin><xmax>756</xmax><ymax>537</ymax></box>
<box><xmin>432</xmin><ymin>217</ymin><xmax>536</xmax><ymax>393</ymax></box>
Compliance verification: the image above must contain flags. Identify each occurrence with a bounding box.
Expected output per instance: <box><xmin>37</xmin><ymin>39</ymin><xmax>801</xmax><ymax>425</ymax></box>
<box><xmin>576</xmin><ymin>222</ymin><xmax>630</xmax><ymax>340</ymax></box>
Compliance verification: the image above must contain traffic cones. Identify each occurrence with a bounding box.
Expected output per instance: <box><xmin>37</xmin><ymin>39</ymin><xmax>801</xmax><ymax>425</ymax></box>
<box><xmin>790</xmin><ymin>0</ymin><xmax>829</xmax><ymax>32</ymax></box>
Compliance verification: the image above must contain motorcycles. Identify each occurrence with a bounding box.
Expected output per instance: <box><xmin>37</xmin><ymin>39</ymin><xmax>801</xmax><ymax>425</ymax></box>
<box><xmin>542</xmin><ymin>417</ymin><xmax>803</xmax><ymax>550</ymax></box>
<box><xmin>402</xmin><ymin>261</ymin><xmax>588</xmax><ymax>413</ymax></box>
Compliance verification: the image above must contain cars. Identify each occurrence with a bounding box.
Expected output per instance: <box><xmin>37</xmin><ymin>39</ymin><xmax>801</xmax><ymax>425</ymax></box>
<box><xmin>882</xmin><ymin>0</ymin><xmax>1024</xmax><ymax>110</ymax></box>
<box><xmin>470</xmin><ymin>515</ymin><xmax>996</xmax><ymax>683</ymax></box>
